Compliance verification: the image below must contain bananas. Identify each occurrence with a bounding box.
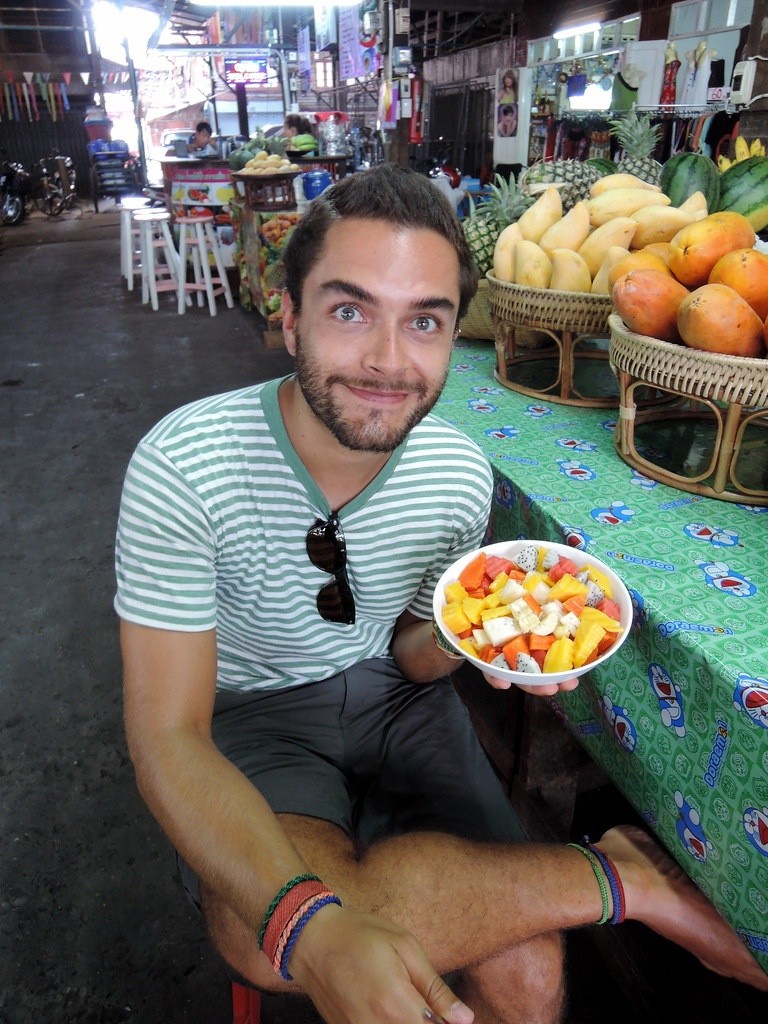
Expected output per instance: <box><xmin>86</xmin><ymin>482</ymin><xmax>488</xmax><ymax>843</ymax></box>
<box><xmin>290</xmin><ymin>133</ymin><xmax>319</xmax><ymax>150</ymax></box>
<box><xmin>718</xmin><ymin>136</ymin><xmax>765</xmax><ymax>172</ymax></box>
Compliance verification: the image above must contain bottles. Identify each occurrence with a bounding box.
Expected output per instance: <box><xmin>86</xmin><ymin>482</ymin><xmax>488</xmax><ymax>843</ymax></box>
<box><xmin>349</xmin><ymin>127</ymin><xmax>383</xmax><ymax>169</ymax></box>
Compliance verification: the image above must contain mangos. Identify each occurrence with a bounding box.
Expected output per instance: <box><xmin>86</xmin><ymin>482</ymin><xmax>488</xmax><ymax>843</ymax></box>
<box><xmin>494</xmin><ymin>174</ymin><xmax>768</xmax><ymax>359</ymax></box>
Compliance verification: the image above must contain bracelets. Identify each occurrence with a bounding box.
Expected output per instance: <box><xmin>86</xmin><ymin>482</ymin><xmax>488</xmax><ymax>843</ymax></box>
<box><xmin>261</xmin><ymin>878</ymin><xmax>334</xmax><ymax>966</ymax></box>
<box><xmin>279</xmin><ymin>896</ymin><xmax>343</xmax><ymax>982</ymax></box>
<box><xmin>255</xmin><ymin>870</ymin><xmax>323</xmax><ymax>952</ymax></box>
<box><xmin>430</xmin><ymin>616</ymin><xmax>467</xmax><ymax>660</ymax></box>
<box><xmin>272</xmin><ymin>890</ymin><xmax>335</xmax><ymax>977</ymax></box>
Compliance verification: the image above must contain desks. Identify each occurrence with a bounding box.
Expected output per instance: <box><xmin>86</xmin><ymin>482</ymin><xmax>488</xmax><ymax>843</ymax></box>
<box><xmin>429</xmin><ymin>334</ymin><xmax>768</xmax><ymax>973</ymax></box>
<box><xmin>229</xmin><ymin>197</ymin><xmax>303</xmax><ymax>330</ymax></box>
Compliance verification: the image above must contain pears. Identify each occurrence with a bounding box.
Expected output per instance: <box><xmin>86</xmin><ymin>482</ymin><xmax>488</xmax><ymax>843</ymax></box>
<box><xmin>237</xmin><ymin>150</ymin><xmax>299</xmax><ymax>174</ymax></box>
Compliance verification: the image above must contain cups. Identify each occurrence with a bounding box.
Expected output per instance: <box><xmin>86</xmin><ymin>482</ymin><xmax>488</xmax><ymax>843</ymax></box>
<box><xmin>302</xmin><ymin>169</ymin><xmax>332</xmax><ymax>201</ymax></box>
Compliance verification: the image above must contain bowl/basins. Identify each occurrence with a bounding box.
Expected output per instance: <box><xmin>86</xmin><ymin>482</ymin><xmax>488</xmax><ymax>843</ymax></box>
<box><xmin>433</xmin><ymin>541</ymin><xmax>634</xmax><ymax>686</ymax></box>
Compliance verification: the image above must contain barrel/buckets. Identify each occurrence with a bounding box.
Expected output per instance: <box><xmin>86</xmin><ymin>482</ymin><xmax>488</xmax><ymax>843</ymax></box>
<box><xmin>82</xmin><ymin>105</ymin><xmax>114</xmax><ymax>142</ymax></box>
<box><xmin>316</xmin><ymin>113</ymin><xmax>346</xmax><ymax>155</ymax></box>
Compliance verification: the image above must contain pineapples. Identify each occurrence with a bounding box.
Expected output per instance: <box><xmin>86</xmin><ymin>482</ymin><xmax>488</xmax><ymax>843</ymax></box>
<box><xmin>460</xmin><ymin>109</ymin><xmax>662</xmax><ymax>280</ymax></box>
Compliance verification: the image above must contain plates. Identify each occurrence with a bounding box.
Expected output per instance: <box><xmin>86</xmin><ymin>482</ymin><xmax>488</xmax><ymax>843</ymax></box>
<box><xmin>230</xmin><ymin>168</ymin><xmax>305</xmax><ymax>182</ymax></box>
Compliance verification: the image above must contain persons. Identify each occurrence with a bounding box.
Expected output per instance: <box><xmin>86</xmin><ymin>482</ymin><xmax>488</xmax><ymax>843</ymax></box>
<box><xmin>606</xmin><ymin>64</ymin><xmax>649</xmax><ymax>117</ymax></box>
<box><xmin>495</xmin><ymin>104</ymin><xmax>519</xmax><ymax>138</ymax></box>
<box><xmin>657</xmin><ymin>41</ymin><xmax>682</xmax><ymax>119</ymax></box>
<box><xmin>282</xmin><ymin>113</ymin><xmax>316</xmax><ymax>158</ymax></box>
<box><xmin>163</xmin><ymin>121</ymin><xmax>219</xmax><ymax>158</ymax></box>
<box><xmin>557</xmin><ymin>71</ymin><xmax>571</xmax><ymax>117</ymax></box>
<box><xmin>111</xmin><ymin>158</ymin><xmax>768</xmax><ymax>1023</ymax></box>
<box><xmin>496</xmin><ymin>69</ymin><xmax>519</xmax><ymax>104</ymax></box>
<box><xmin>678</xmin><ymin>41</ymin><xmax>717</xmax><ymax>120</ymax></box>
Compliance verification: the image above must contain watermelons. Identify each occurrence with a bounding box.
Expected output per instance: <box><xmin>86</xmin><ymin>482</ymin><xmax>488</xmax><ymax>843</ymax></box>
<box><xmin>229</xmin><ymin>143</ymin><xmax>262</xmax><ymax>169</ymax></box>
<box><xmin>583</xmin><ymin>152</ymin><xmax>768</xmax><ymax>233</ymax></box>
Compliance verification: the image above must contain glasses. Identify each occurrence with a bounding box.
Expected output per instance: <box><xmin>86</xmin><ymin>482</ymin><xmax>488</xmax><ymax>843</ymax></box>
<box><xmin>306</xmin><ymin>510</ymin><xmax>356</xmax><ymax>626</ymax></box>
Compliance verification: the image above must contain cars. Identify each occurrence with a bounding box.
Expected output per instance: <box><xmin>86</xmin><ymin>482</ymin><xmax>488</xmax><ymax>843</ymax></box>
<box><xmin>159</xmin><ymin>127</ymin><xmax>200</xmax><ymax>175</ymax></box>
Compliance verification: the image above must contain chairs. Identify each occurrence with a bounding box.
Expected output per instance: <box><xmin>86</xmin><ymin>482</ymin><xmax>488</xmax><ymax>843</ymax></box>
<box><xmin>88</xmin><ymin>150</ymin><xmax>149</xmax><ymax>213</ymax></box>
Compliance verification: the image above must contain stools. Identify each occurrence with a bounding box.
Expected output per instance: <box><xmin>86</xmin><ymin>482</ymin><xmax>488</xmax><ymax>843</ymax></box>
<box><xmin>175</xmin><ymin>214</ymin><xmax>235</xmax><ymax>318</ymax></box>
<box><xmin>118</xmin><ymin>195</ymin><xmax>193</xmax><ymax>310</ymax></box>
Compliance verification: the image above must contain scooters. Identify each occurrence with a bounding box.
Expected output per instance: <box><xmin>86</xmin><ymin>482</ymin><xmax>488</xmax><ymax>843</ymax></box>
<box><xmin>1</xmin><ymin>147</ymin><xmax>76</xmax><ymax>226</ymax></box>
